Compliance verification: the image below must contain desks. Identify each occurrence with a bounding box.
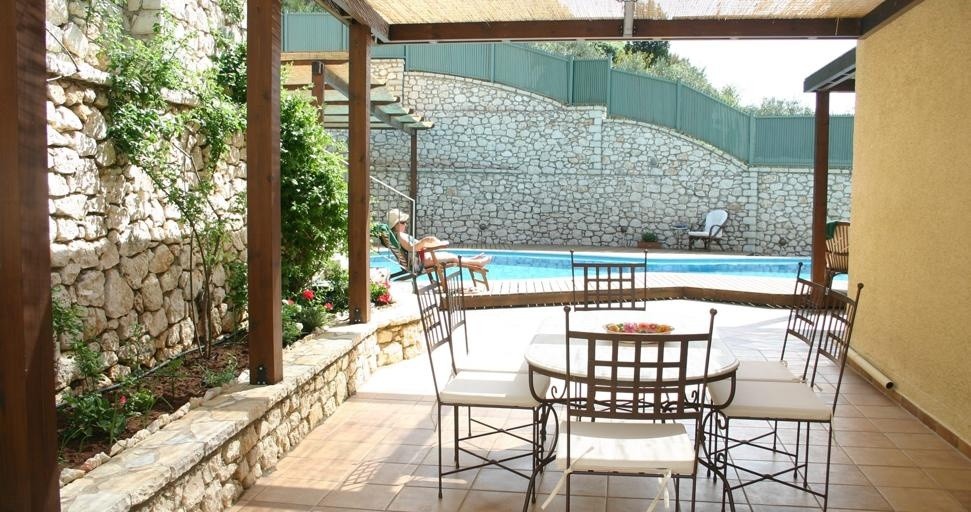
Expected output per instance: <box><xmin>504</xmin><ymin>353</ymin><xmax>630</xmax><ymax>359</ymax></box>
<box><xmin>516</xmin><ymin>300</ymin><xmax>743</xmax><ymax>512</ymax></box>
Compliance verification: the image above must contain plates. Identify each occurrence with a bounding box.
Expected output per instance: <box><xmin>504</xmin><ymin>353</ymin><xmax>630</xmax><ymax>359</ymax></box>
<box><xmin>603</xmin><ymin>322</ymin><xmax>674</xmax><ymax>342</ymax></box>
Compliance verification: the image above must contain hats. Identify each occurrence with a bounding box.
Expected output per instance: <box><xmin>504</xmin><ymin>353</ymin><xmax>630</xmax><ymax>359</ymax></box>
<box><xmin>387</xmin><ymin>209</ymin><xmax>409</xmax><ymax>229</ymax></box>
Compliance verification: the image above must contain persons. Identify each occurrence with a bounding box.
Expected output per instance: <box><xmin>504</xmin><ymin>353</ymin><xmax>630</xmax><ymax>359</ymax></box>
<box><xmin>385</xmin><ymin>208</ymin><xmax>494</xmax><ymax>270</ymax></box>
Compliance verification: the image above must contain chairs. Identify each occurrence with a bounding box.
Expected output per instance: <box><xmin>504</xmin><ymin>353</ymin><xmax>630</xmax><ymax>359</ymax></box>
<box><xmin>407</xmin><ymin>253</ymin><xmax>552</xmax><ymax>505</ymax></box>
<box><xmin>704</xmin><ymin>260</ymin><xmax>862</xmax><ymax>511</ymax></box>
<box><xmin>566</xmin><ymin>246</ymin><xmax>652</xmax><ymax>434</ymax></box>
<box><xmin>552</xmin><ymin>303</ymin><xmax>719</xmax><ymax>512</ymax></box>
<box><xmin>374</xmin><ymin>218</ymin><xmax>494</xmax><ymax>293</ymax></box>
<box><xmin>686</xmin><ymin>209</ymin><xmax>730</xmax><ymax>252</ymax></box>
